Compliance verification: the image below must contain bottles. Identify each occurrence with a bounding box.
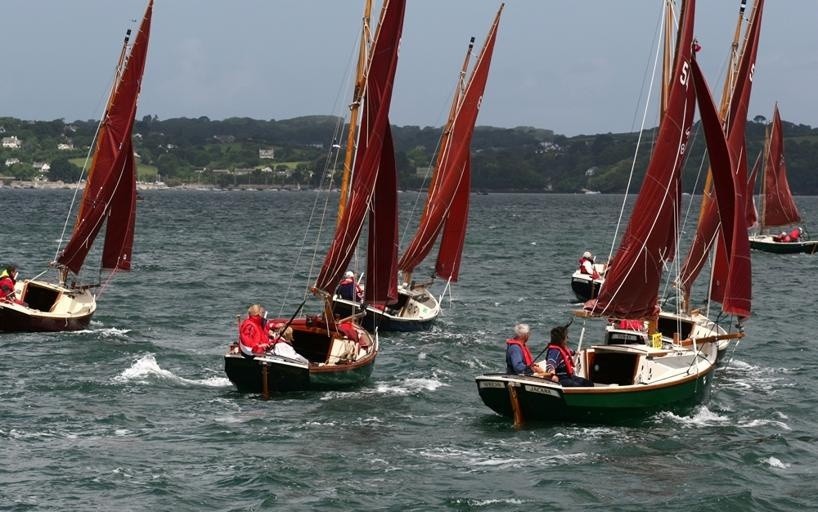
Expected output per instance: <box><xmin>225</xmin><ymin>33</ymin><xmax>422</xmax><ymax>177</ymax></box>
<box><xmin>341</xmin><ymin>270</ymin><xmax>355</xmax><ymax>279</ymax></box>
<box><xmin>583</xmin><ymin>252</ymin><xmax>592</xmax><ymax>258</ymax></box>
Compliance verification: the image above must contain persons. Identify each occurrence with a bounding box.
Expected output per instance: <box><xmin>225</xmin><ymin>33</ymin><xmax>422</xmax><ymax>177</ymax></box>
<box><xmin>239</xmin><ymin>305</ymin><xmax>286</xmax><ymax>358</ymax></box>
<box><xmin>777</xmin><ymin>231</ymin><xmax>790</xmax><ymax>242</ymax></box>
<box><xmin>0</xmin><ymin>265</ymin><xmax>29</xmax><ymax>307</ymax></box>
<box><xmin>335</xmin><ymin>271</ymin><xmax>363</xmax><ymax>304</ymax></box>
<box><xmin>546</xmin><ymin>326</ymin><xmax>595</xmax><ymax>387</ymax></box>
<box><xmin>789</xmin><ymin>227</ymin><xmax>804</xmax><ymax>243</ymax></box>
<box><xmin>578</xmin><ymin>251</ymin><xmax>603</xmax><ymax>280</ymax></box>
<box><xmin>505</xmin><ymin>323</ymin><xmax>558</xmax><ymax>384</ymax></box>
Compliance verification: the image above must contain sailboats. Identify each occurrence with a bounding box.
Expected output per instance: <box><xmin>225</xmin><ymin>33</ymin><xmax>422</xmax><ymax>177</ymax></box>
<box><xmin>746</xmin><ymin>100</ymin><xmax>818</xmax><ymax>254</ymax></box>
<box><xmin>1</xmin><ymin>1</ymin><xmax>155</xmax><ymax>334</ymax></box>
<box><xmin>329</xmin><ymin>1</ymin><xmax>506</xmax><ymax>335</ymax></box>
<box><xmin>475</xmin><ymin>1</ymin><xmax>765</xmax><ymax>422</ymax></box>
<box><xmin>222</xmin><ymin>0</ymin><xmax>380</xmax><ymax>398</ymax></box>
